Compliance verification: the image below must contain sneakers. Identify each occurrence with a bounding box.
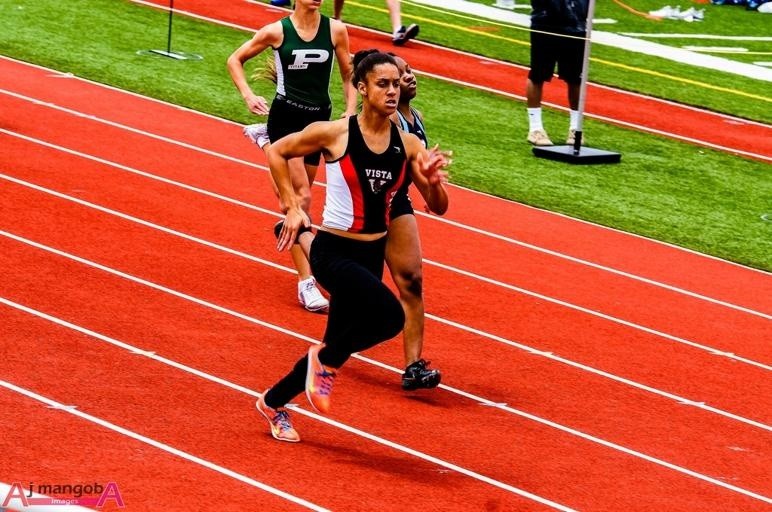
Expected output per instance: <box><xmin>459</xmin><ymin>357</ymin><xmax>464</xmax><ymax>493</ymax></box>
<box><xmin>255</xmin><ymin>388</ymin><xmax>301</xmax><ymax>443</ymax></box>
<box><xmin>272</xmin><ymin>218</ymin><xmax>309</xmax><ymax>242</ymax></box>
<box><xmin>567</xmin><ymin>130</ymin><xmax>583</xmax><ymax>145</ymax></box>
<box><xmin>527</xmin><ymin>128</ymin><xmax>552</xmax><ymax>146</ymax></box>
<box><xmin>392</xmin><ymin>22</ymin><xmax>420</xmax><ymax>44</ymax></box>
<box><xmin>243</xmin><ymin>122</ymin><xmax>267</xmax><ymax>144</ymax></box>
<box><xmin>649</xmin><ymin>5</ymin><xmax>705</xmax><ymax>21</ymax></box>
<box><xmin>402</xmin><ymin>358</ymin><xmax>442</xmax><ymax>391</ymax></box>
<box><xmin>298</xmin><ymin>277</ymin><xmax>330</xmax><ymax>313</ymax></box>
<box><xmin>305</xmin><ymin>343</ymin><xmax>337</xmax><ymax>415</ymax></box>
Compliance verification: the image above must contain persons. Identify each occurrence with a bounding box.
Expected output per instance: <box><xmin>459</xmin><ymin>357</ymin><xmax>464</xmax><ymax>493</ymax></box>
<box><xmin>526</xmin><ymin>0</ymin><xmax>590</xmax><ymax>146</ymax></box>
<box><xmin>255</xmin><ymin>48</ymin><xmax>449</xmax><ymax>443</ymax></box>
<box><xmin>274</xmin><ymin>51</ymin><xmax>454</xmax><ymax>391</ymax></box>
<box><xmin>334</xmin><ymin>0</ymin><xmax>420</xmax><ymax>47</ymax></box>
<box><xmin>226</xmin><ymin>0</ymin><xmax>357</xmax><ymax>314</ymax></box>
